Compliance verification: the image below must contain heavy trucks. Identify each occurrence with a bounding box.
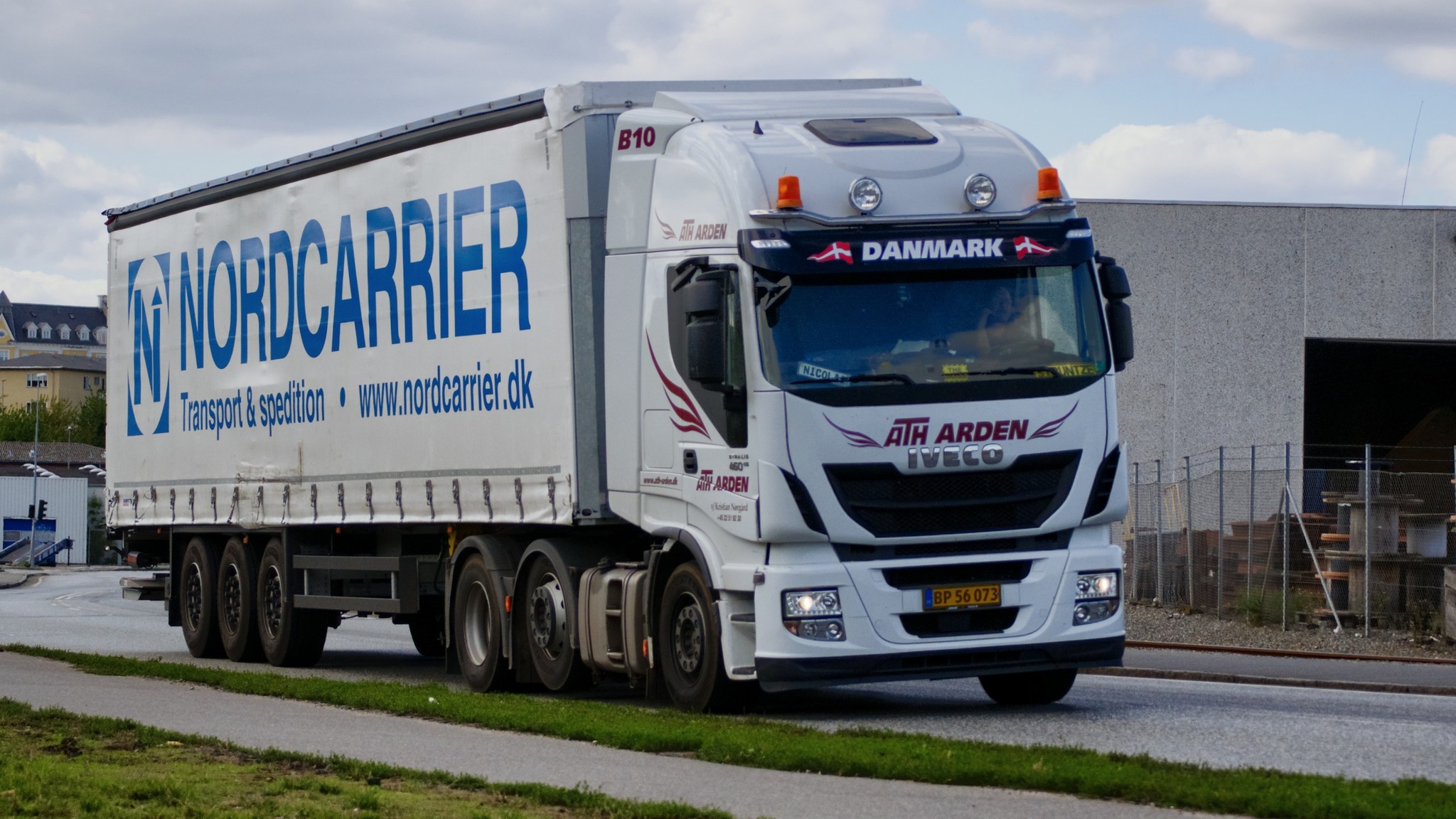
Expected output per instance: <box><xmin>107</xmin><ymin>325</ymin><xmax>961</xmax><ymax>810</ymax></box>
<box><xmin>104</xmin><ymin>75</ymin><xmax>1138</xmax><ymax>717</ymax></box>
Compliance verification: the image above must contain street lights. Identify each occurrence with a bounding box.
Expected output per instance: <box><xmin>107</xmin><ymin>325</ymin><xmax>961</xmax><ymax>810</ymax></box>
<box><xmin>68</xmin><ymin>426</ymin><xmax>73</xmax><ymax>470</ymax></box>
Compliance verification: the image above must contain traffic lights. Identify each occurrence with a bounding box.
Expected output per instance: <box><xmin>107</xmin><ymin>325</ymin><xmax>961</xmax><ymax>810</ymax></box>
<box><xmin>38</xmin><ymin>499</ymin><xmax>48</xmax><ymax>520</ymax></box>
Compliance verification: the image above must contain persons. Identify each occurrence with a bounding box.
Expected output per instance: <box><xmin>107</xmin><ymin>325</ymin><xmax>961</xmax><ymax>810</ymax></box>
<box><xmin>974</xmin><ymin>287</ymin><xmax>1035</xmax><ymax>359</ymax></box>
